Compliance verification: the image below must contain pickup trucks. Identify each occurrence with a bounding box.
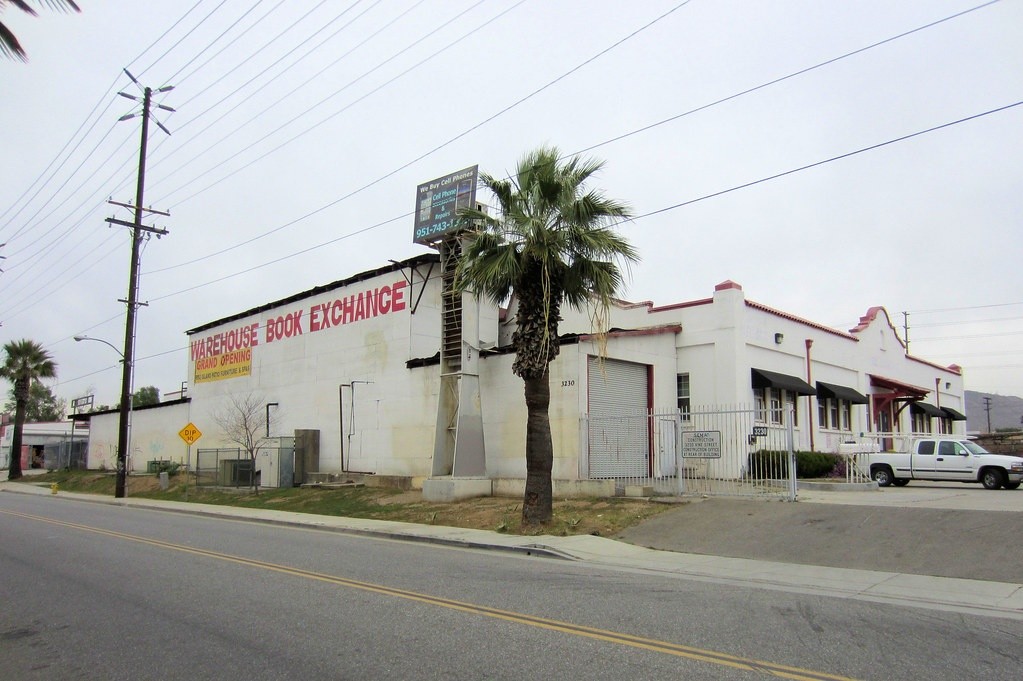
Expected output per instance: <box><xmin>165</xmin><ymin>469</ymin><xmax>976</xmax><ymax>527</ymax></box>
<box><xmin>850</xmin><ymin>436</ymin><xmax>1023</xmax><ymax>490</ymax></box>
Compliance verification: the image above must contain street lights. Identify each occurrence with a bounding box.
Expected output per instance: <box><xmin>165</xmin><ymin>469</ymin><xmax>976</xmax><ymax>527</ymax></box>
<box><xmin>74</xmin><ymin>335</ymin><xmax>133</xmax><ymax>498</ymax></box>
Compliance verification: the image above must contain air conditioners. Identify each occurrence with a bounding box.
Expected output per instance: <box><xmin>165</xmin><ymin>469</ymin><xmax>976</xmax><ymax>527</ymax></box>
<box><xmin>219</xmin><ymin>459</ymin><xmax>254</xmax><ymax>487</ymax></box>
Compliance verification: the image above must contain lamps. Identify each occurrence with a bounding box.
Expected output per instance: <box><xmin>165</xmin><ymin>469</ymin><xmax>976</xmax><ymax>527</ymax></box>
<box><xmin>775</xmin><ymin>333</ymin><xmax>784</xmax><ymax>343</ymax></box>
<box><xmin>946</xmin><ymin>382</ymin><xmax>952</xmax><ymax>389</ymax></box>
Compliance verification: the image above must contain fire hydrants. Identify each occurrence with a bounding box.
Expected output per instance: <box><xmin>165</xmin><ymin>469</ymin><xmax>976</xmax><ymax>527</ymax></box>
<box><xmin>51</xmin><ymin>482</ymin><xmax>59</xmax><ymax>494</ymax></box>
